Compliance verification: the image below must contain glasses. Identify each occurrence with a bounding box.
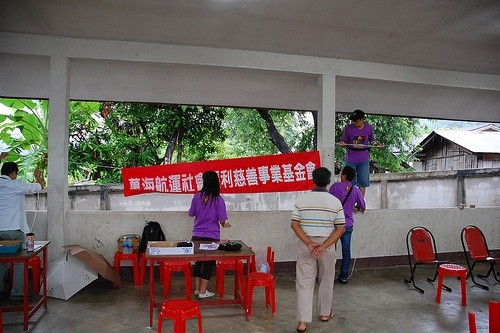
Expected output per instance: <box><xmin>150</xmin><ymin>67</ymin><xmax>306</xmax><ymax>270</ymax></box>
<box><xmin>339</xmin><ymin>172</ymin><xmax>342</xmax><ymax>175</ymax></box>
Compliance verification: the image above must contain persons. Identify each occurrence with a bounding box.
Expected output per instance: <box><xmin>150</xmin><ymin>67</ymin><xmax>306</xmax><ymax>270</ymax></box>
<box><xmin>291</xmin><ymin>167</ymin><xmax>346</xmax><ymax>333</ymax></box>
<box><xmin>328</xmin><ymin>166</ymin><xmax>365</xmax><ymax>283</ymax></box>
<box><xmin>188</xmin><ymin>171</ymin><xmax>231</xmax><ymax>299</ymax></box>
<box><xmin>339</xmin><ymin>110</ymin><xmax>381</xmax><ymax>210</ymax></box>
<box><xmin>0</xmin><ymin>162</ymin><xmax>44</xmax><ymax>300</ymax></box>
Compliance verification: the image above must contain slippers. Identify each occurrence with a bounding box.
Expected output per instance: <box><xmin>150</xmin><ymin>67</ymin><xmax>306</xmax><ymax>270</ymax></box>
<box><xmin>297</xmin><ymin>323</ymin><xmax>308</xmax><ymax>333</ymax></box>
<box><xmin>320</xmin><ymin>309</ymin><xmax>334</xmax><ymax>321</ymax></box>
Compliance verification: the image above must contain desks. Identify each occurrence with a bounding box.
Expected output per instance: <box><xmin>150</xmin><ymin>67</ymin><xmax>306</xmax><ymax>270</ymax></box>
<box><xmin>144</xmin><ymin>239</ymin><xmax>255</xmax><ymax>327</ymax></box>
<box><xmin>0</xmin><ymin>240</ymin><xmax>51</xmax><ymax>333</ymax></box>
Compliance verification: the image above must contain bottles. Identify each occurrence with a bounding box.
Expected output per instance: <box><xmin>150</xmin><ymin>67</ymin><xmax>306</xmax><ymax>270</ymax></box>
<box><xmin>123</xmin><ymin>237</ymin><xmax>132</xmax><ymax>254</ymax></box>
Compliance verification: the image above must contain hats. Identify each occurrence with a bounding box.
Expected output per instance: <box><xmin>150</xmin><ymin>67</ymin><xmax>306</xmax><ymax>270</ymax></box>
<box><xmin>349</xmin><ymin>110</ymin><xmax>365</xmax><ymax>121</ymax></box>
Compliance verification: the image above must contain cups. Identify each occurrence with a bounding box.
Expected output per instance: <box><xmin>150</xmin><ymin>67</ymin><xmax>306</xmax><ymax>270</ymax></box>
<box><xmin>25</xmin><ymin>233</ymin><xmax>35</xmax><ymax>252</ymax></box>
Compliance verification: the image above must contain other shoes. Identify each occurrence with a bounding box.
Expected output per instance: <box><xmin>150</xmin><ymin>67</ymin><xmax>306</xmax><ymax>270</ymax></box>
<box><xmin>194</xmin><ymin>291</ymin><xmax>199</xmax><ymax>295</ymax></box>
<box><xmin>338</xmin><ymin>278</ymin><xmax>348</xmax><ymax>284</ymax></box>
<box><xmin>198</xmin><ymin>290</ymin><xmax>215</xmax><ymax>298</ymax></box>
<box><xmin>12</xmin><ymin>295</ymin><xmax>31</xmax><ymax>300</ymax></box>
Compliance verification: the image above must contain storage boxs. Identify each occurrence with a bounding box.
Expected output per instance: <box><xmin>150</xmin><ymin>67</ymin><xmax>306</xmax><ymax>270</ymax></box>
<box><xmin>146</xmin><ymin>241</ymin><xmax>194</xmax><ymax>255</ymax></box>
<box><xmin>0</xmin><ymin>241</ymin><xmax>24</xmax><ymax>254</ymax></box>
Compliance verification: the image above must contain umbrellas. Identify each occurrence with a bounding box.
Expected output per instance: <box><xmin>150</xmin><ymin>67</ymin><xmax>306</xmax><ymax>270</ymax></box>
<box><xmin>39</xmin><ymin>254</ymin><xmax>99</xmax><ymax>301</ymax></box>
<box><xmin>61</xmin><ymin>244</ymin><xmax>122</xmax><ymax>287</ymax></box>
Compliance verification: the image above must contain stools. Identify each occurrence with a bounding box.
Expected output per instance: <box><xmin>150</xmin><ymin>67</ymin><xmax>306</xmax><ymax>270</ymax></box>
<box><xmin>112</xmin><ymin>247</ymin><xmax>277</xmax><ymax>333</ymax></box>
<box><xmin>10</xmin><ymin>254</ymin><xmax>41</xmax><ymax>294</ymax></box>
<box><xmin>467</xmin><ymin>301</ymin><xmax>500</xmax><ymax>333</ymax></box>
<box><xmin>436</xmin><ymin>263</ymin><xmax>468</xmax><ymax>306</ymax></box>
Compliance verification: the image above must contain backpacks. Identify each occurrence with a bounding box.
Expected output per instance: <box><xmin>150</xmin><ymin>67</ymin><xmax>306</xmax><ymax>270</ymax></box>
<box><xmin>138</xmin><ymin>222</ymin><xmax>165</xmax><ymax>253</ymax></box>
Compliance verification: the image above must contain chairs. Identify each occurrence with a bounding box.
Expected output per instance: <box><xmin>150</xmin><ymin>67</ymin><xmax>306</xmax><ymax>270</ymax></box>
<box><xmin>457</xmin><ymin>225</ymin><xmax>500</xmax><ymax>291</ymax></box>
<box><xmin>403</xmin><ymin>226</ymin><xmax>452</xmax><ymax>294</ymax></box>
